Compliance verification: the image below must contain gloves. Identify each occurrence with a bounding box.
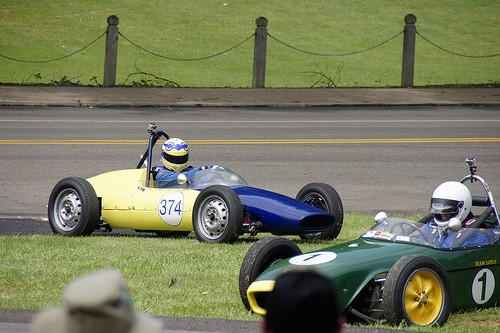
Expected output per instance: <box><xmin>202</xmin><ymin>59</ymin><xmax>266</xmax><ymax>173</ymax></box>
<box><xmin>211</xmin><ymin>164</ymin><xmax>224</xmax><ymax>170</ymax></box>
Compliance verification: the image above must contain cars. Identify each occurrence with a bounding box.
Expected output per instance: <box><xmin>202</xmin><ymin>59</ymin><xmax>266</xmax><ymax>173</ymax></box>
<box><xmin>46</xmin><ymin>121</ymin><xmax>345</xmax><ymax>245</ymax></box>
<box><xmin>238</xmin><ymin>157</ymin><xmax>500</xmax><ymax>331</ymax></box>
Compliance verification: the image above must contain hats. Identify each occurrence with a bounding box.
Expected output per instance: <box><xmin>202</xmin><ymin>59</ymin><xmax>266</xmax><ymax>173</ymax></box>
<box><xmin>32</xmin><ymin>269</ymin><xmax>163</xmax><ymax>333</ymax></box>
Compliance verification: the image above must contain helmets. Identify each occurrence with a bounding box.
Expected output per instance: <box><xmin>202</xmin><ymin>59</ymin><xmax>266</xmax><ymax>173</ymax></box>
<box><xmin>430</xmin><ymin>180</ymin><xmax>472</xmax><ymax>227</ymax></box>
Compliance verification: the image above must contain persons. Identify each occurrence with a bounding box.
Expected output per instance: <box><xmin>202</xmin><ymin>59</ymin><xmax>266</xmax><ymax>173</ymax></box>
<box><xmin>156</xmin><ymin>138</ymin><xmax>224</xmax><ymax>189</ymax></box>
<box><xmin>29</xmin><ymin>271</ymin><xmax>165</xmax><ymax>333</ymax></box>
<box><xmin>419</xmin><ymin>181</ymin><xmax>495</xmax><ymax>250</ymax></box>
<box><xmin>260</xmin><ymin>271</ymin><xmax>346</xmax><ymax>333</ymax></box>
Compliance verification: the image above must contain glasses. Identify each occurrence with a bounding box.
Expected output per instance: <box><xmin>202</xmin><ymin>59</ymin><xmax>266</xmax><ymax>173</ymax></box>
<box><xmin>161</xmin><ymin>137</ymin><xmax>189</xmax><ymax>173</ymax></box>
